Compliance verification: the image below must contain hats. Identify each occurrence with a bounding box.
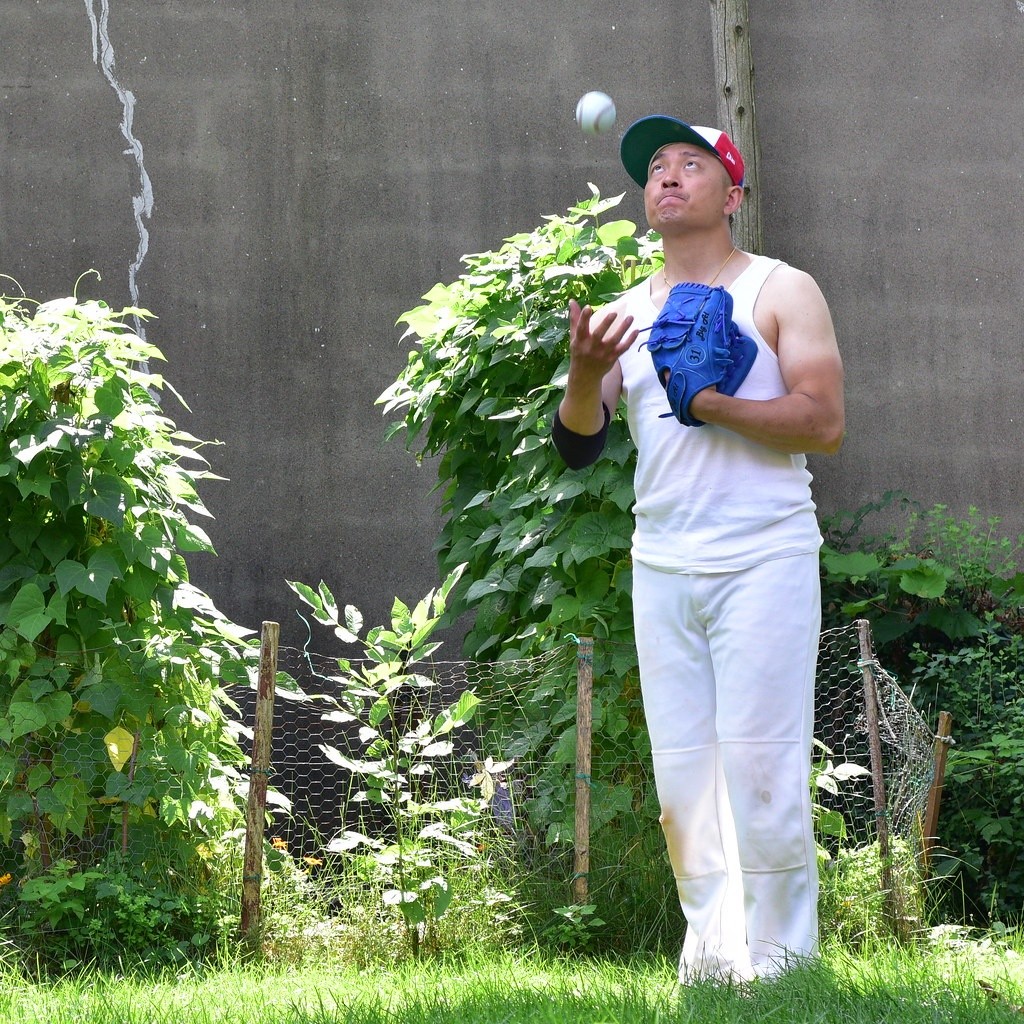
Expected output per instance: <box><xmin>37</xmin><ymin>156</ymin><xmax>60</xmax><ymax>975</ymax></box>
<box><xmin>620</xmin><ymin>115</ymin><xmax>743</xmax><ymax>189</ymax></box>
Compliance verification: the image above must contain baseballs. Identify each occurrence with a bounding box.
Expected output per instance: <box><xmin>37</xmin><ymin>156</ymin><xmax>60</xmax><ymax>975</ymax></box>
<box><xmin>576</xmin><ymin>91</ymin><xmax>618</xmax><ymax>135</ymax></box>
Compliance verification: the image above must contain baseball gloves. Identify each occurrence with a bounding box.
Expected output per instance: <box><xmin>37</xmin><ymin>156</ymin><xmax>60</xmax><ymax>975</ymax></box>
<box><xmin>647</xmin><ymin>282</ymin><xmax>758</xmax><ymax>428</ymax></box>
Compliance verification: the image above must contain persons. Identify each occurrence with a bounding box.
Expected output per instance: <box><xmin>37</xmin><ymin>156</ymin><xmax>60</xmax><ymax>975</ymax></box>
<box><xmin>550</xmin><ymin>113</ymin><xmax>846</xmax><ymax>990</ymax></box>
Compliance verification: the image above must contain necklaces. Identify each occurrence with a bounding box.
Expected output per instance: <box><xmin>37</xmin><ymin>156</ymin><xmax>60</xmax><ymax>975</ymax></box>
<box><xmin>663</xmin><ymin>244</ymin><xmax>738</xmax><ymax>290</ymax></box>
